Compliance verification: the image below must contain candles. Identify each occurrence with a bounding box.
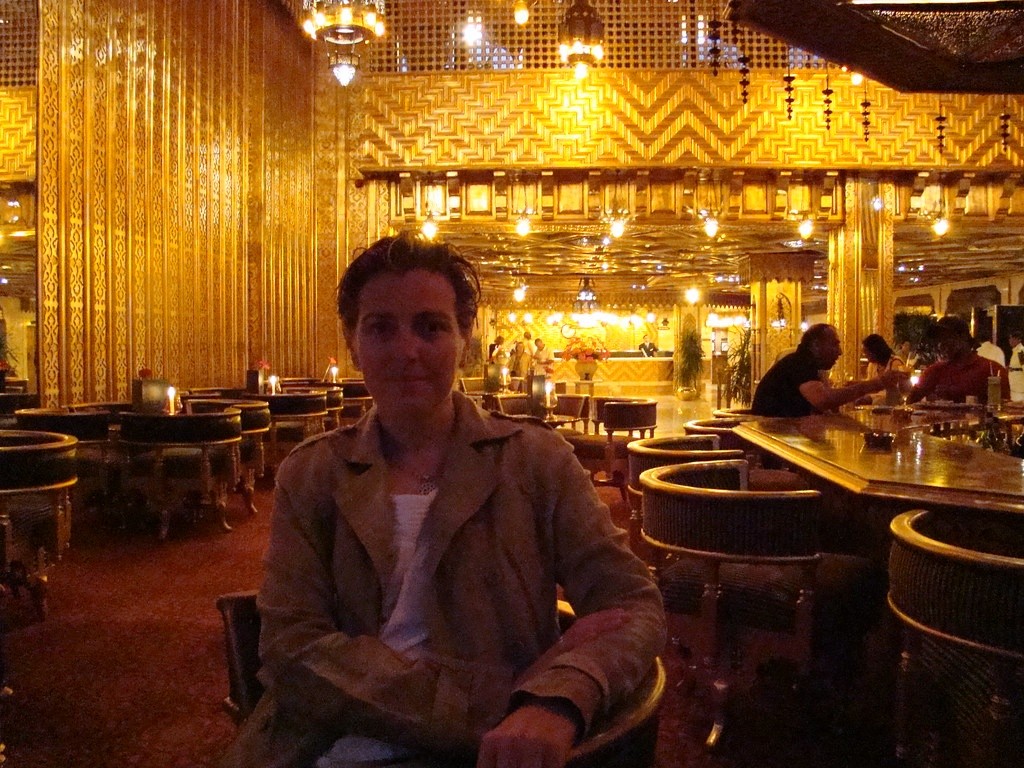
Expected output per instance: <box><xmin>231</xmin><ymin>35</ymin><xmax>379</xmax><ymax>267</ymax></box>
<box><xmin>331</xmin><ymin>367</ymin><xmax>338</xmax><ymax>383</ymax></box>
<box><xmin>168</xmin><ymin>386</ymin><xmax>176</xmax><ymax>415</ymax></box>
<box><xmin>270</xmin><ymin>376</ymin><xmax>277</xmax><ymax>396</ymax></box>
<box><xmin>545</xmin><ymin>381</ymin><xmax>552</xmax><ymax>407</ymax></box>
<box><xmin>502</xmin><ymin>368</ymin><xmax>508</xmax><ymax>386</ymax></box>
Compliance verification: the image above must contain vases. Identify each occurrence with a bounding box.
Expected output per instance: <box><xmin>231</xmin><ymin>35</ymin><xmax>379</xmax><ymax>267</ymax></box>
<box><xmin>575</xmin><ymin>359</ymin><xmax>598</xmax><ymax>381</ymax></box>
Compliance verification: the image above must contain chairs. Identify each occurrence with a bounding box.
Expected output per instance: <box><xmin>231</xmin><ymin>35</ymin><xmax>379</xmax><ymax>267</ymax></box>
<box><xmin>216</xmin><ymin>588</ymin><xmax>667</xmax><ymax>767</ymax></box>
<box><xmin>1</xmin><ymin>375</ymin><xmax>373</xmax><ymax>620</ymax></box>
<box><xmin>458</xmin><ymin>376</ymin><xmax>1024</xmax><ymax>768</ymax></box>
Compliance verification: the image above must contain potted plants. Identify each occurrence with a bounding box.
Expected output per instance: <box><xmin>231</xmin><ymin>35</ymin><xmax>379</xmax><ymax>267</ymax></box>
<box><xmin>673</xmin><ymin>329</ymin><xmax>706</xmax><ymax>401</ymax></box>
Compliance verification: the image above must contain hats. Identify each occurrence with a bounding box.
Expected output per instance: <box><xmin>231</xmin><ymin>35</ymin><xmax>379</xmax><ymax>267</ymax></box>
<box><xmin>927</xmin><ymin>317</ymin><xmax>969</xmax><ymax>338</ymax></box>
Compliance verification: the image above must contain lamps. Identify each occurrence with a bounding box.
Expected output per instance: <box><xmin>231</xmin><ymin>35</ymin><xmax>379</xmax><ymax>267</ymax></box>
<box><xmin>555</xmin><ymin>0</ymin><xmax>604</xmax><ymax>80</ymax></box>
<box><xmin>300</xmin><ymin>0</ymin><xmax>386</xmax><ymax>87</ymax></box>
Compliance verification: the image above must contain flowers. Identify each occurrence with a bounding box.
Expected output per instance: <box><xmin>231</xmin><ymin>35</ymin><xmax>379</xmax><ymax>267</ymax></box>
<box><xmin>138</xmin><ymin>368</ymin><xmax>152</xmax><ymax>386</ymax></box>
<box><xmin>249</xmin><ymin>358</ymin><xmax>269</xmax><ymax>371</ymax></box>
<box><xmin>561</xmin><ymin>335</ymin><xmax>610</xmax><ymax>363</ymax></box>
<box><xmin>322</xmin><ymin>355</ymin><xmax>338</xmax><ymax>381</ymax></box>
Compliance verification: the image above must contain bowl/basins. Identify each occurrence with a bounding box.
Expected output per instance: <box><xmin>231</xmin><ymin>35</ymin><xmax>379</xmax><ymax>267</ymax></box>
<box><xmin>862</xmin><ymin>432</ymin><xmax>895</xmax><ymax>447</ymax></box>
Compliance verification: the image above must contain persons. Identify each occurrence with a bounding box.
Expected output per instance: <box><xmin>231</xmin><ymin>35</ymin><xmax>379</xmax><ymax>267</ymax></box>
<box><xmin>223</xmin><ymin>230</ymin><xmax>668</xmax><ymax>768</ymax></box>
<box><xmin>893</xmin><ymin>330</ymin><xmax>1024</xmax><ymax>372</ymax></box>
<box><xmin>844</xmin><ymin>333</ymin><xmax>907</xmax><ymax>405</ymax></box>
<box><xmin>752</xmin><ymin>324</ymin><xmax>911</xmax><ymax>417</ymax></box>
<box><xmin>489</xmin><ymin>332</ymin><xmax>554</xmax><ymax>377</ymax></box>
<box><xmin>905</xmin><ymin>317</ymin><xmax>1011</xmax><ymax>404</ymax></box>
<box><xmin>638</xmin><ymin>334</ymin><xmax>660</xmax><ymax>357</ymax></box>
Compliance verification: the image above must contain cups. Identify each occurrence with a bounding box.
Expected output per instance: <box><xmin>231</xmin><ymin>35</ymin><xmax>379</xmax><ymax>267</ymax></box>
<box><xmin>844</xmin><ymin>369</ymin><xmax>853</xmax><ymax>382</ymax></box>
<box><xmin>987</xmin><ymin>377</ymin><xmax>1001</xmax><ymax>412</ymax></box>
<box><xmin>898</xmin><ymin>366</ymin><xmax>913</xmax><ymax>386</ymax></box>
<box><xmin>966</xmin><ymin>395</ymin><xmax>978</xmax><ymax>403</ymax></box>
